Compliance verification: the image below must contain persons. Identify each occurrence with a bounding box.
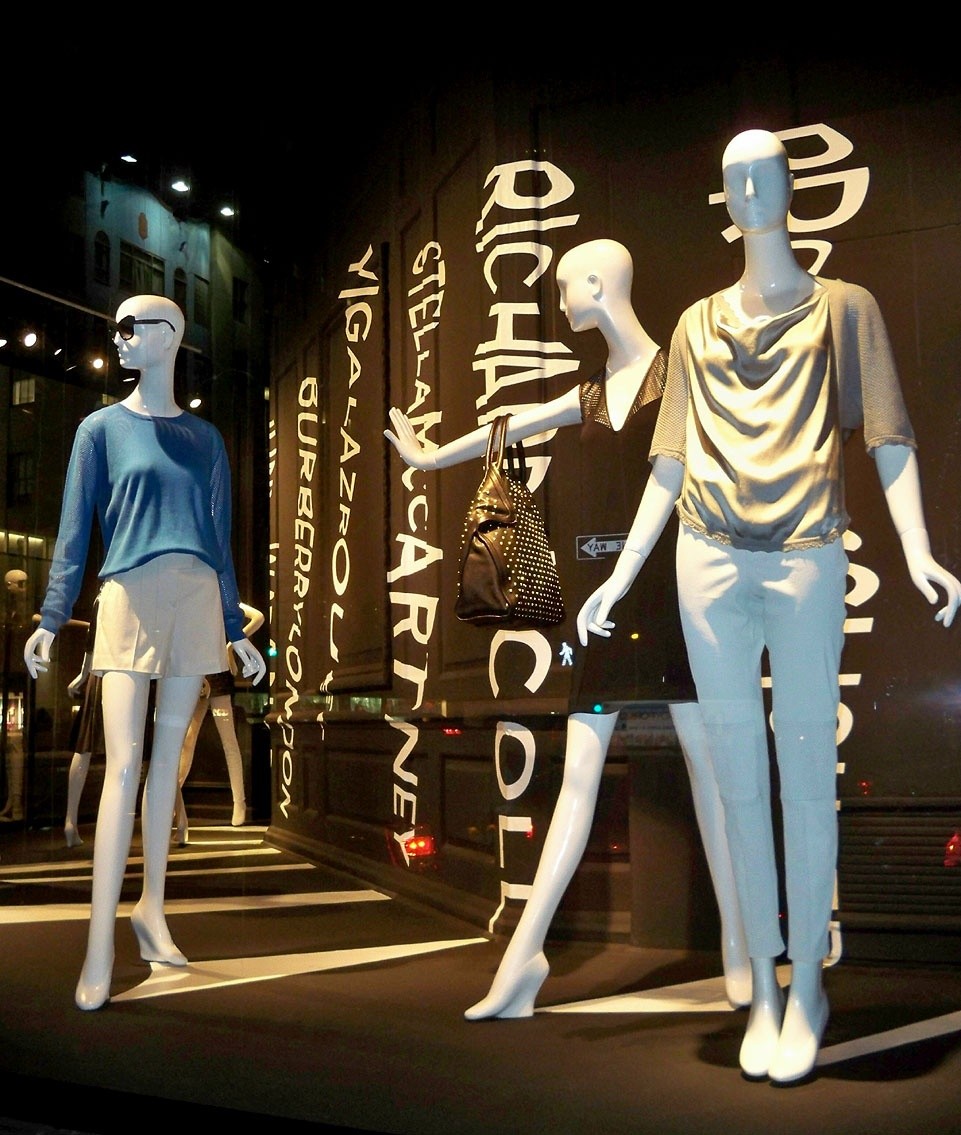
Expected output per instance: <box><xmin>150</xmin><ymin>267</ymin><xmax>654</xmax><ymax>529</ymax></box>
<box><xmin>0</xmin><ymin>570</ymin><xmax>265</xmax><ymax>848</ymax></box>
<box><xmin>574</xmin><ymin>131</ymin><xmax>961</xmax><ymax>1080</ymax></box>
<box><xmin>382</xmin><ymin>237</ymin><xmax>753</xmax><ymax>1018</ymax></box>
<box><xmin>23</xmin><ymin>295</ymin><xmax>267</xmax><ymax>1009</ymax></box>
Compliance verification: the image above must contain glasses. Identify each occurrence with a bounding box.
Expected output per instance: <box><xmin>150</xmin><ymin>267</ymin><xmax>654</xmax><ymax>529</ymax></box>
<box><xmin>107</xmin><ymin>315</ymin><xmax>176</xmax><ymax>340</ymax></box>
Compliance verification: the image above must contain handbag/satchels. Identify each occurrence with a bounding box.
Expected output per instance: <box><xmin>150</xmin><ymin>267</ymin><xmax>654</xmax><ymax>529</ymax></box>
<box><xmin>453</xmin><ymin>413</ymin><xmax>565</xmax><ymax>629</ymax></box>
